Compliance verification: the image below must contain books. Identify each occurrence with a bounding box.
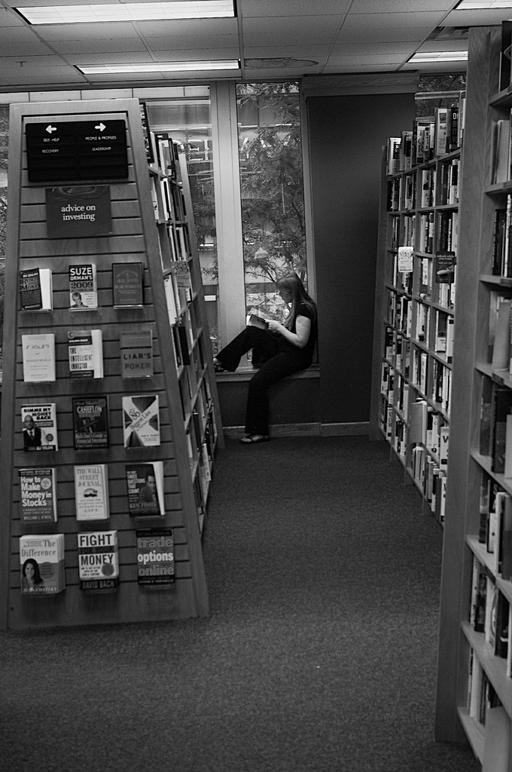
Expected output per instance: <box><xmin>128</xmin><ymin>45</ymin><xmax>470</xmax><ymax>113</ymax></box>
<box><xmin>19</xmin><ymin>262</ymin><xmax>145</xmax><ymax>313</ymax></box>
<box><xmin>246</xmin><ymin>307</ymin><xmax>271</xmax><ymax>330</ymax></box>
<box><xmin>22</xmin><ymin>329</ymin><xmax>154</xmax><ymax>382</ymax></box>
<box><xmin>19</xmin><ymin>528</ymin><xmax>176</xmax><ymax>594</ymax></box>
<box><xmin>21</xmin><ymin>395</ymin><xmax>160</xmax><ymax>453</ymax></box>
<box><xmin>139</xmin><ymin>101</ymin><xmax>218</xmax><ymax>542</ymax></box>
<box><xmin>467</xmin><ymin>20</ymin><xmax>511</xmax><ymax>728</ymax></box>
<box><xmin>378</xmin><ymin>89</ymin><xmax>468</xmax><ymax>523</ymax></box>
<box><xmin>19</xmin><ymin>461</ymin><xmax>166</xmax><ymax>523</ymax></box>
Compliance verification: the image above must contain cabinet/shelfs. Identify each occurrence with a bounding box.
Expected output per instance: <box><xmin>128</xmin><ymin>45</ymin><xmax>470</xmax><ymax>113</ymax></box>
<box><xmin>435</xmin><ymin>20</ymin><xmax>512</xmax><ymax>772</ymax></box>
<box><xmin>371</xmin><ymin>98</ymin><xmax>471</xmax><ymax>528</ymax></box>
<box><xmin>1</xmin><ymin>98</ymin><xmax>226</xmax><ymax>633</ymax></box>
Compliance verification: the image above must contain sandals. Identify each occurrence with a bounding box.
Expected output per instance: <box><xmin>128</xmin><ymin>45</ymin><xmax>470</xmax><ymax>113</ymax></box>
<box><xmin>240</xmin><ymin>433</ymin><xmax>269</xmax><ymax>444</ymax></box>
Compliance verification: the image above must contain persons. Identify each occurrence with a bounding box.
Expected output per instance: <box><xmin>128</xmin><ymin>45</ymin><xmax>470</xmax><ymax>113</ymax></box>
<box><xmin>213</xmin><ymin>272</ymin><xmax>317</xmax><ymax>445</ymax></box>
